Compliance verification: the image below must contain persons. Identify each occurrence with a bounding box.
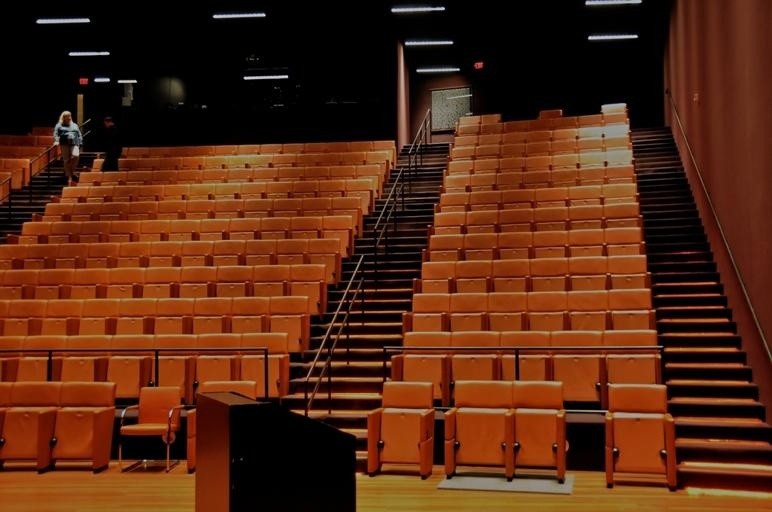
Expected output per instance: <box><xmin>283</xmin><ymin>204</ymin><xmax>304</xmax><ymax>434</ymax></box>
<box><xmin>100</xmin><ymin>113</ymin><xmax>123</xmax><ymax>172</ymax></box>
<box><xmin>53</xmin><ymin>109</ymin><xmax>84</xmax><ymax>187</ymax></box>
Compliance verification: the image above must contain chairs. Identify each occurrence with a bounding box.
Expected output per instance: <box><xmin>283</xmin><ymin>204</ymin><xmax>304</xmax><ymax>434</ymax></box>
<box><xmin>290</xmin><ymin>142</ymin><xmax>366</xmax><ymax>352</ymax></box>
<box><xmin>368</xmin><ymin>103</ymin><xmax>678</xmax><ymax>493</ymax></box>
<box><xmin>0</xmin><ymin>128</ymin><xmax>290</xmax><ymax>474</ymax></box>
